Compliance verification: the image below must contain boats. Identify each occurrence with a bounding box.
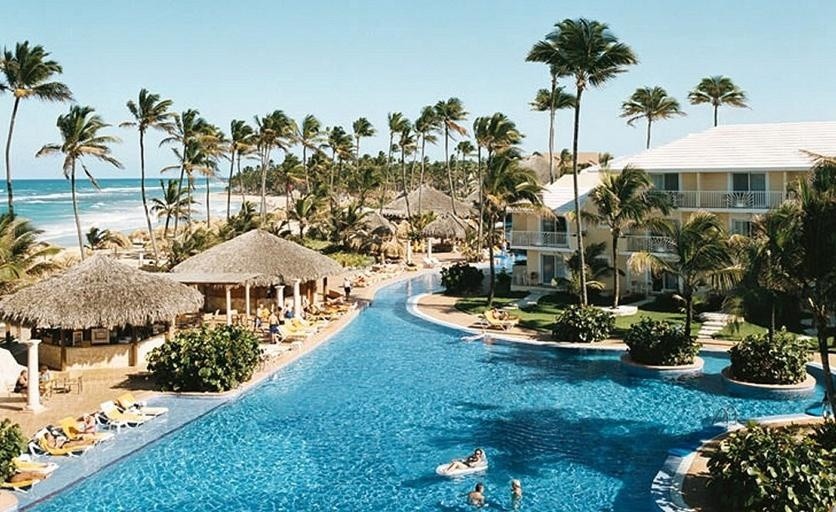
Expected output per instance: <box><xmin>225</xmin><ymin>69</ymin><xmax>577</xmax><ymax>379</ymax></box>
<box><xmin>433</xmin><ymin>457</ymin><xmax>490</xmax><ymax>477</ymax></box>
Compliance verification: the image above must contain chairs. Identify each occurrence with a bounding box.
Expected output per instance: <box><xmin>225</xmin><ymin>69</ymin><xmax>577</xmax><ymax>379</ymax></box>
<box><xmin>0</xmin><ymin>392</ymin><xmax>169</xmax><ymax>494</ymax></box>
<box><xmin>345</xmin><ymin>256</ymin><xmax>450</xmax><ymax>288</ymax></box>
<box><xmin>480</xmin><ymin>308</ymin><xmax>521</xmax><ymax>332</ymax></box>
<box><xmin>180</xmin><ymin>288</ymin><xmax>360</xmax><ymax>347</ymax></box>
<box><xmin>6</xmin><ymin>370</ymin><xmax>84</xmax><ymax>401</ymax></box>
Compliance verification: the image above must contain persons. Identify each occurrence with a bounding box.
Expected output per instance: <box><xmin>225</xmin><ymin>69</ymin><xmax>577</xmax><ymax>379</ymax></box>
<box><xmin>252</xmin><ymin>293</ymin><xmax>344</xmax><ymax>341</ymax></box>
<box><xmin>44</xmin><ymin>432</ymin><xmax>95</xmax><ymax>449</ymax></box>
<box><xmin>342</xmin><ymin>276</ymin><xmax>353</xmax><ymax>301</ymax></box>
<box><xmin>38</xmin><ymin>365</ymin><xmax>52</xmax><ymax>402</ymax></box>
<box><xmin>82</xmin><ymin>415</ymin><xmax>99</xmax><ymax>436</ymax></box>
<box><xmin>510</xmin><ymin>478</ymin><xmax>523</xmax><ymax>511</ymax></box>
<box><xmin>12</xmin><ymin>369</ymin><xmax>29</xmax><ymax>402</ymax></box>
<box><xmin>3</xmin><ymin>466</ymin><xmax>47</xmax><ymax>483</ymax></box>
<box><xmin>442</xmin><ymin>447</ymin><xmax>483</xmax><ymax>473</ymax></box>
<box><xmin>464</xmin><ymin>482</ymin><xmax>485</xmax><ymax>506</ymax></box>
<box><xmin>492</xmin><ymin>307</ymin><xmax>520</xmax><ymax>320</ymax></box>
